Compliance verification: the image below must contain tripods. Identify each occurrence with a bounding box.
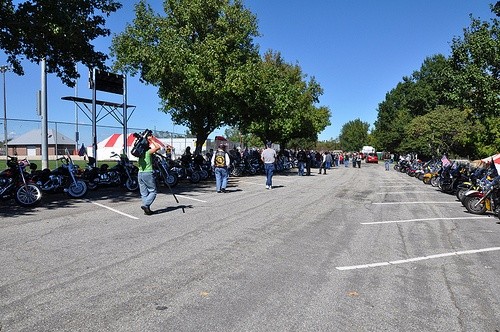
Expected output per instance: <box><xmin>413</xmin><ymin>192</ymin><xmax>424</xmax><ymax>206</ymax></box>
<box><xmin>154</xmin><ymin>152</ymin><xmax>183</xmax><ymax>204</ymax></box>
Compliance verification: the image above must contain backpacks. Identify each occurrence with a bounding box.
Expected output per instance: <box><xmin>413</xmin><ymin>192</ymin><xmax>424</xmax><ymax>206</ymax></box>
<box><xmin>214</xmin><ymin>150</ymin><xmax>226</xmax><ymax>167</ymax></box>
<box><xmin>131</xmin><ymin>129</ymin><xmax>153</xmax><ymax>157</ymax></box>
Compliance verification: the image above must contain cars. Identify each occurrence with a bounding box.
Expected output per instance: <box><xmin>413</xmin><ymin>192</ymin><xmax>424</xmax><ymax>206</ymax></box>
<box><xmin>352</xmin><ymin>153</ymin><xmax>366</xmax><ymax>161</ymax></box>
<box><xmin>365</xmin><ymin>153</ymin><xmax>379</xmax><ymax>164</ymax></box>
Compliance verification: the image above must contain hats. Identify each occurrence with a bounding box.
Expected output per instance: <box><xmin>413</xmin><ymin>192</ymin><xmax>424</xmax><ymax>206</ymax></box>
<box><xmin>267</xmin><ymin>142</ymin><xmax>271</xmax><ymax>146</ymax></box>
<box><xmin>218</xmin><ymin>143</ymin><xmax>226</xmax><ymax>148</ymax></box>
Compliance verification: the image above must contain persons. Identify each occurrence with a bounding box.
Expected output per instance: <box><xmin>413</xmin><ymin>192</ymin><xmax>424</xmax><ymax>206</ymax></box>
<box><xmin>261</xmin><ymin>142</ymin><xmax>278</xmax><ymax>189</ymax></box>
<box><xmin>384</xmin><ymin>151</ymin><xmax>391</xmax><ymax>170</ymax></box>
<box><xmin>183</xmin><ymin>147</ymin><xmax>191</xmax><ymax>163</ymax></box>
<box><xmin>297</xmin><ymin>148</ymin><xmax>306</xmax><ymax>175</ymax></box>
<box><xmin>352</xmin><ymin>151</ymin><xmax>361</xmax><ymax>168</ymax></box>
<box><xmin>331</xmin><ymin>153</ymin><xmax>339</xmax><ymax>167</ymax></box>
<box><xmin>339</xmin><ymin>152</ymin><xmax>344</xmax><ymax>164</ymax></box>
<box><xmin>138</xmin><ymin>137</ymin><xmax>160</xmax><ymax>213</ymax></box>
<box><xmin>326</xmin><ymin>152</ymin><xmax>332</xmax><ymax>169</ymax></box>
<box><xmin>206</xmin><ymin>148</ymin><xmax>214</xmax><ymax>161</ymax></box>
<box><xmin>344</xmin><ymin>153</ymin><xmax>349</xmax><ymax>167</ymax></box>
<box><xmin>359</xmin><ymin>152</ymin><xmax>366</xmax><ymax>163</ymax></box>
<box><xmin>211</xmin><ymin>144</ymin><xmax>231</xmax><ymax>192</ymax></box>
<box><xmin>390</xmin><ymin>152</ymin><xmax>400</xmax><ymax>164</ymax></box>
<box><xmin>318</xmin><ymin>152</ymin><xmax>327</xmax><ymax>174</ymax></box>
<box><xmin>305</xmin><ymin>150</ymin><xmax>313</xmax><ymax>175</ymax></box>
<box><xmin>229</xmin><ymin>148</ymin><xmax>250</xmax><ymax>159</ymax></box>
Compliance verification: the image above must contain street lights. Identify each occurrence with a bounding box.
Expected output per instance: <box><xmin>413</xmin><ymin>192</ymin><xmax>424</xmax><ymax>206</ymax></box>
<box><xmin>0</xmin><ymin>65</ymin><xmax>10</xmax><ymax>168</ymax></box>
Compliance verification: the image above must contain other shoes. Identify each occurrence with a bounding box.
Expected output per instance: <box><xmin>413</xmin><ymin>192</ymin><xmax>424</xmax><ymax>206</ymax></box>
<box><xmin>141</xmin><ymin>205</ymin><xmax>153</xmax><ymax>215</ymax></box>
<box><xmin>269</xmin><ymin>186</ymin><xmax>271</xmax><ymax>190</ymax></box>
<box><xmin>222</xmin><ymin>188</ymin><xmax>226</xmax><ymax>193</ymax></box>
<box><xmin>266</xmin><ymin>185</ymin><xmax>269</xmax><ymax>189</ymax></box>
<box><xmin>218</xmin><ymin>191</ymin><xmax>223</xmax><ymax>193</ymax></box>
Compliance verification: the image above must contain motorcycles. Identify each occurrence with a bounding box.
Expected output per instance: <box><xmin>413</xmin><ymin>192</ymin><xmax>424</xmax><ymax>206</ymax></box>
<box><xmin>29</xmin><ymin>148</ymin><xmax>87</xmax><ymax>198</ymax></box>
<box><xmin>0</xmin><ymin>155</ymin><xmax>45</xmax><ymax>207</ymax></box>
<box><xmin>80</xmin><ymin>151</ymin><xmax>139</xmax><ymax>191</ymax></box>
<box><xmin>394</xmin><ymin>154</ymin><xmax>500</xmax><ymax>214</ymax></box>
<box><xmin>138</xmin><ymin>154</ymin><xmax>291</xmax><ymax>189</ymax></box>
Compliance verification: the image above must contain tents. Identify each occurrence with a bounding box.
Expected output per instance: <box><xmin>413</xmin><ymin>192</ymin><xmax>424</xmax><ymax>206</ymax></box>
<box><xmin>481</xmin><ymin>152</ymin><xmax>500</xmax><ymax>176</ymax></box>
<box><xmin>87</xmin><ymin>133</ymin><xmax>176</xmax><ymax>162</ymax></box>
<box><xmin>471</xmin><ymin>159</ymin><xmax>481</xmax><ymax>167</ymax></box>
